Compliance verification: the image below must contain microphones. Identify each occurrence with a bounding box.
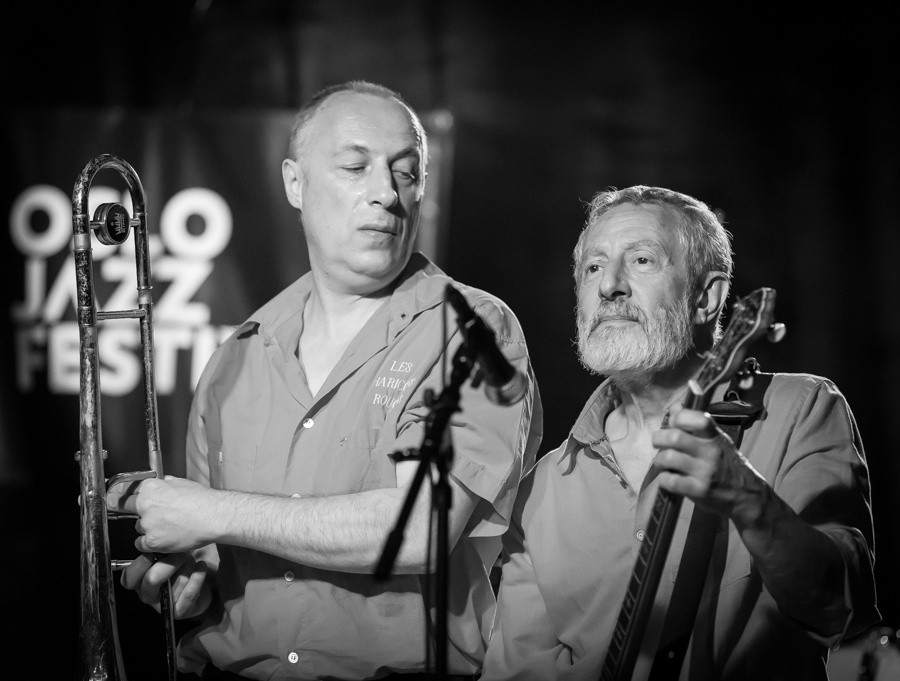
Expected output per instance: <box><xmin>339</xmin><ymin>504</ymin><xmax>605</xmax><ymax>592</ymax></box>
<box><xmin>447</xmin><ymin>285</ymin><xmax>530</xmax><ymax>408</ymax></box>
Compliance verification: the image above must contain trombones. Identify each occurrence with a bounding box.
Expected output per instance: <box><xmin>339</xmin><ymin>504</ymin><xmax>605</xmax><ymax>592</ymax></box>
<box><xmin>70</xmin><ymin>154</ymin><xmax>179</xmax><ymax>681</ymax></box>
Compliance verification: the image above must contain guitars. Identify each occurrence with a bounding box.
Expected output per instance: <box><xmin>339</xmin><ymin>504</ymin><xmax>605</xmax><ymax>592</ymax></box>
<box><xmin>595</xmin><ymin>286</ymin><xmax>790</xmax><ymax>681</ymax></box>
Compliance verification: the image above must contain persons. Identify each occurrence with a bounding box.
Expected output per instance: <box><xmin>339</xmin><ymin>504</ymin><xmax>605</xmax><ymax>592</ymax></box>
<box><xmin>480</xmin><ymin>185</ymin><xmax>883</xmax><ymax>681</ymax></box>
<box><xmin>97</xmin><ymin>79</ymin><xmax>544</xmax><ymax>681</ymax></box>
